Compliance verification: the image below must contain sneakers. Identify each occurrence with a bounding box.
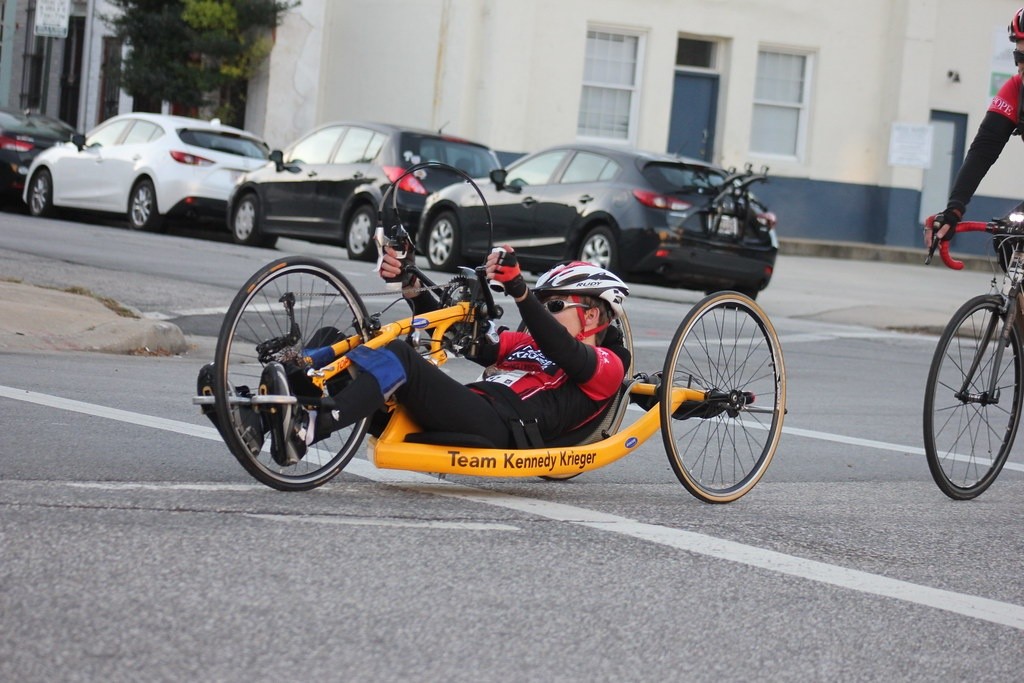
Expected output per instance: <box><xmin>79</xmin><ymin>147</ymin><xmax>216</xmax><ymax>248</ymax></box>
<box><xmin>197</xmin><ymin>361</ymin><xmax>265</xmax><ymax>459</ymax></box>
<box><xmin>257</xmin><ymin>361</ymin><xmax>317</xmax><ymax>466</ymax></box>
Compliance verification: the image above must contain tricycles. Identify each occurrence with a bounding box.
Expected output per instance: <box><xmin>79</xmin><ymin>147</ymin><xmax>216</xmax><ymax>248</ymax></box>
<box><xmin>193</xmin><ymin>161</ymin><xmax>789</xmax><ymax>504</ymax></box>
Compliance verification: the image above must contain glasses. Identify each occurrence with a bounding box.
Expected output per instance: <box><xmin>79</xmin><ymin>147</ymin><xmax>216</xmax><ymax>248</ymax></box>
<box><xmin>544</xmin><ymin>299</ymin><xmax>590</xmax><ymax>313</ymax></box>
<box><xmin>1013</xmin><ymin>51</ymin><xmax>1024</xmax><ymax>63</ymax></box>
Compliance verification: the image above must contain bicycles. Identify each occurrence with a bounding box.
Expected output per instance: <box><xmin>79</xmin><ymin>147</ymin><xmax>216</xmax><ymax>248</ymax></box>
<box><xmin>920</xmin><ymin>208</ymin><xmax>1024</xmax><ymax>500</ymax></box>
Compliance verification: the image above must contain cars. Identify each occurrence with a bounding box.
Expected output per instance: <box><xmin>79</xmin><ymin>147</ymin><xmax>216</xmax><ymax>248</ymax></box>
<box><xmin>21</xmin><ymin>108</ymin><xmax>274</xmax><ymax>230</ymax></box>
<box><xmin>228</xmin><ymin>118</ymin><xmax>501</xmax><ymax>259</ymax></box>
<box><xmin>1</xmin><ymin>100</ymin><xmax>87</xmax><ymax>205</ymax></box>
<box><xmin>416</xmin><ymin>141</ymin><xmax>779</xmax><ymax>308</ymax></box>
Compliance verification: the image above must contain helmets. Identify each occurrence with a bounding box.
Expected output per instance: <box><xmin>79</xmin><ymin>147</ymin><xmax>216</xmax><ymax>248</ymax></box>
<box><xmin>1007</xmin><ymin>7</ymin><xmax>1024</xmax><ymax>42</ymax></box>
<box><xmin>529</xmin><ymin>260</ymin><xmax>630</xmax><ymax>296</ymax></box>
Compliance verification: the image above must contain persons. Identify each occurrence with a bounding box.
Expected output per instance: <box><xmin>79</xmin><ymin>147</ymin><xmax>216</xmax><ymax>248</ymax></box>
<box><xmin>195</xmin><ymin>231</ymin><xmax>629</xmax><ymax>464</ymax></box>
<box><xmin>924</xmin><ymin>6</ymin><xmax>1024</xmax><ymax>244</ymax></box>
